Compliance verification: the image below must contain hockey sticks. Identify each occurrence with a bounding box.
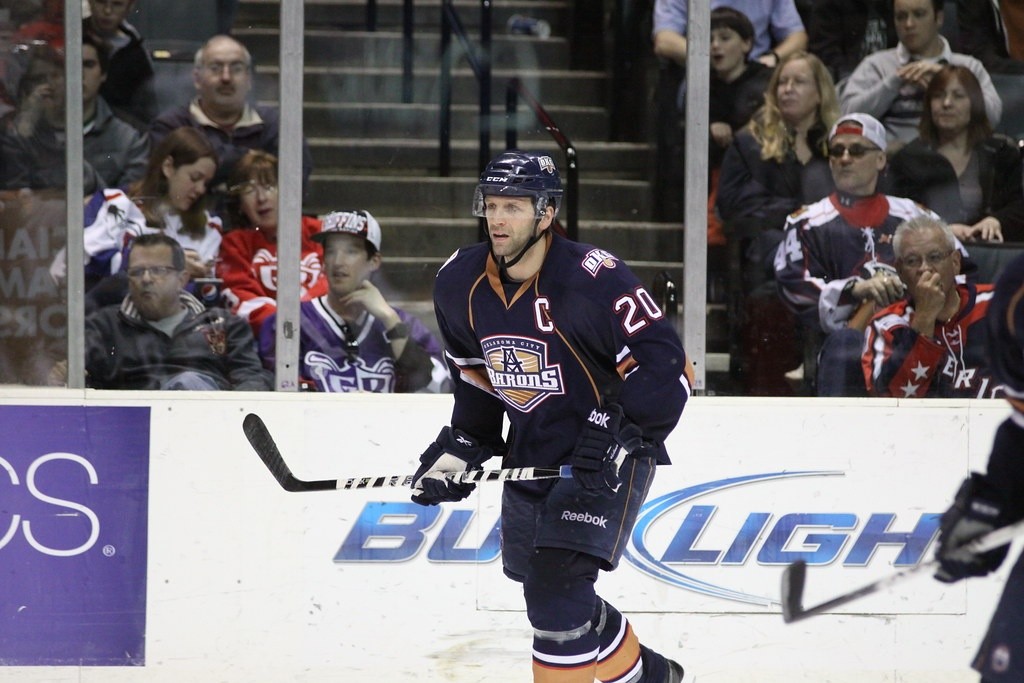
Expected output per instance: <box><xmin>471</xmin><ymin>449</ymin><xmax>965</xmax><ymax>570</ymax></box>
<box><xmin>241</xmin><ymin>409</ymin><xmax>617</xmax><ymax>493</ymax></box>
<box><xmin>779</xmin><ymin>520</ymin><xmax>1023</xmax><ymax>626</ymax></box>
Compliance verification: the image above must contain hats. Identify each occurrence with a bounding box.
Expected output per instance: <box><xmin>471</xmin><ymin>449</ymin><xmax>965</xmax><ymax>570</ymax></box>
<box><xmin>312</xmin><ymin>210</ymin><xmax>381</xmax><ymax>252</ymax></box>
<box><xmin>829</xmin><ymin>112</ymin><xmax>886</xmax><ymax>152</ymax></box>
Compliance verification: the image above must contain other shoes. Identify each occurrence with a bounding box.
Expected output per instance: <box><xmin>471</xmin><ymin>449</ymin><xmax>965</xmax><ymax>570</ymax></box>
<box><xmin>637</xmin><ymin>644</ymin><xmax>684</xmax><ymax>683</ymax></box>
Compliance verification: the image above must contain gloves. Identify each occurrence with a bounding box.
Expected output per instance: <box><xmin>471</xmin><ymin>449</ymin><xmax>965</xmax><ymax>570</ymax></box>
<box><xmin>411</xmin><ymin>426</ymin><xmax>493</xmax><ymax>507</ymax></box>
<box><xmin>560</xmin><ymin>403</ymin><xmax>642</xmax><ymax>499</ymax></box>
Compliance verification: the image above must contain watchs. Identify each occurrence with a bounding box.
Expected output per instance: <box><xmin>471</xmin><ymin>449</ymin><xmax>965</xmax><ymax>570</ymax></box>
<box><xmin>380</xmin><ymin>321</ymin><xmax>408</xmax><ymax>343</ymax></box>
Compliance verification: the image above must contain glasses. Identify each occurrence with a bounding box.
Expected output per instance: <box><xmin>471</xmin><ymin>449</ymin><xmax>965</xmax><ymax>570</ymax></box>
<box><xmin>206</xmin><ymin>63</ymin><xmax>250</xmax><ymax>75</ymax></box>
<box><xmin>346</xmin><ymin>320</ymin><xmax>359</xmax><ymax>363</ymax></box>
<box><xmin>126</xmin><ymin>265</ymin><xmax>179</xmax><ymax>279</ymax></box>
<box><xmin>897</xmin><ymin>248</ymin><xmax>954</xmax><ymax>267</ymax></box>
<box><xmin>828</xmin><ymin>144</ymin><xmax>879</xmax><ymax>157</ymax></box>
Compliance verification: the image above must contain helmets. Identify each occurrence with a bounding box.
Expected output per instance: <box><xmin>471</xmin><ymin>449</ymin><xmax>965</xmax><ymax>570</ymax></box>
<box><xmin>480</xmin><ymin>150</ymin><xmax>565</xmax><ymax>197</ymax></box>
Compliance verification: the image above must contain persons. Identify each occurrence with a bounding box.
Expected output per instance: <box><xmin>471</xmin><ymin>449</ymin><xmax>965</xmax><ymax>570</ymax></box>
<box><xmin>138</xmin><ymin>34</ymin><xmax>309</xmax><ymax>230</ymax></box>
<box><xmin>217</xmin><ymin>150</ymin><xmax>330</xmax><ymax>335</ymax></box>
<box><xmin>411</xmin><ymin>152</ymin><xmax>694</xmax><ymax>683</ymax></box>
<box><xmin>840</xmin><ymin>0</ymin><xmax>1003</xmax><ymax>144</ymax></box>
<box><xmin>259</xmin><ymin>208</ymin><xmax>457</xmax><ymax>393</ymax></box>
<box><xmin>652</xmin><ymin>0</ymin><xmax>808</xmax><ymax>61</ymax></box>
<box><xmin>936</xmin><ymin>252</ymin><xmax>1024</xmax><ymax>683</ymax></box>
<box><xmin>774</xmin><ymin>112</ymin><xmax>977</xmax><ymax>396</ymax></box>
<box><xmin>862</xmin><ymin>212</ymin><xmax>997</xmax><ymax>397</ymax></box>
<box><xmin>888</xmin><ymin>64</ymin><xmax>1023</xmax><ymax>243</ymax></box>
<box><xmin>715</xmin><ymin>48</ymin><xmax>843</xmax><ymax>397</ymax></box>
<box><xmin>50</xmin><ymin>127</ymin><xmax>222</xmax><ymax>306</ymax></box>
<box><xmin>671</xmin><ymin>7</ymin><xmax>773</xmax><ymax>298</ymax></box>
<box><xmin>0</xmin><ymin>0</ymin><xmax>154</xmax><ymax>194</ymax></box>
<box><xmin>47</xmin><ymin>234</ymin><xmax>276</xmax><ymax>391</ymax></box>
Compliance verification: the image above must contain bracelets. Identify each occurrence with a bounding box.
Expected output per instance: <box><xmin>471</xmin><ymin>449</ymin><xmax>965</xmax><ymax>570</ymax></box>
<box><xmin>760</xmin><ymin>50</ymin><xmax>780</xmax><ymax>64</ymax></box>
